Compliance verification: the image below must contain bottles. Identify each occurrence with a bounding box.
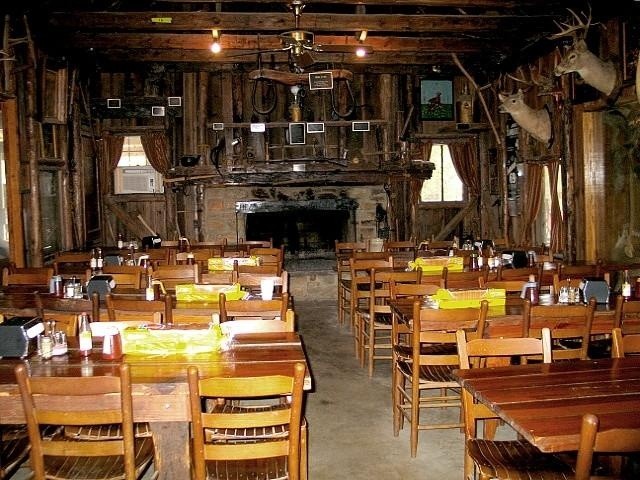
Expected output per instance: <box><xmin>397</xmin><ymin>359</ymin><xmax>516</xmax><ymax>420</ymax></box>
<box><xmin>90</xmin><ymin>248</ymin><xmax>105</xmax><ymax>271</ymax></box>
<box><xmin>469</xmin><ymin>253</ymin><xmax>484</xmax><ymax>270</ymax></box>
<box><xmin>145</xmin><ymin>275</ymin><xmax>155</xmax><ymax>302</ymax></box>
<box><xmin>187</xmin><ymin>252</ymin><xmax>196</xmax><ymax>264</ymax></box>
<box><xmin>124</xmin><ymin>255</ymin><xmax>150</xmax><ymax>267</ymax></box>
<box><xmin>559</xmin><ymin>286</ymin><xmax>580</xmax><ymax>305</ymax></box>
<box><xmin>39</xmin><ymin>330</ymin><xmax>69</xmax><ymax>360</ymax></box>
<box><xmin>77</xmin><ymin>312</ymin><xmax>94</xmax><ymax>358</ymax></box>
<box><xmin>487</xmin><ymin>254</ymin><xmax>502</xmax><ymax>270</ymax></box>
<box><xmin>527</xmin><ymin>250</ymin><xmax>535</xmax><ymax>267</ymax></box>
<box><xmin>463</xmin><ymin>242</ymin><xmax>475</xmax><ymax>251</ymax></box>
<box><xmin>635</xmin><ymin>276</ymin><xmax>640</xmax><ymax>299</ymax></box>
<box><xmin>48</xmin><ymin>274</ymin><xmax>84</xmax><ymax>299</ymax></box>
<box><xmin>621</xmin><ymin>270</ymin><xmax>632</xmax><ymax>301</ymax></box>
<box><xmin>116</xmin><ymin>233</ymin><xmax>139</xmax><ymax>250</ymax></box>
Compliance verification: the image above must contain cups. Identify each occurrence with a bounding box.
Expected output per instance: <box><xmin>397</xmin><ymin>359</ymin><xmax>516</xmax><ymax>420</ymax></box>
<box><xmin>261</xmin><ymin>277</ymin><xmax>275</xmax><ymax>302</ymax></box>
<box><xmin>540</xmin><ymin>295</ymin><xmax>552</xmax><ymax>304</ymax></box>
<box><xmin>176</xmin><ymin>258</ymin><xmax>187</xmax><ymax>265</ymax></box>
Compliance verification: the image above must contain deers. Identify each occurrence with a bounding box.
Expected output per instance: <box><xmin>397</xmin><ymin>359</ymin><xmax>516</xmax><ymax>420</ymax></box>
<box><xmin>546</xmin><ymin>5</ymin><xmax>618</xmax><ymax>100</ymax></box>
<box><xmin>490</xmin><ymin>59</ymin><xmax>554</xmax><ymax>149</ymax></box>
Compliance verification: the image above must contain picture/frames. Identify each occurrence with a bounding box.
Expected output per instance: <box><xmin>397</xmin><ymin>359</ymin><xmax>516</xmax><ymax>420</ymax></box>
<box><xmin>417</xmin><ymin>76</ymin><xmax>456</xmax><ymax>123</ymax></box>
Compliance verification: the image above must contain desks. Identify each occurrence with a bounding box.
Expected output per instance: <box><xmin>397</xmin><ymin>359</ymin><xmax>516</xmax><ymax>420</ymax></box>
<box><xmin>450</xmin><ymin>356</ymin><xmax>638</xmax><ymax>457</ymax></box>
<box><xmin>0</xmin><ymin>330</ymin><xmax>312</xmax><ymax>461</ymax></box>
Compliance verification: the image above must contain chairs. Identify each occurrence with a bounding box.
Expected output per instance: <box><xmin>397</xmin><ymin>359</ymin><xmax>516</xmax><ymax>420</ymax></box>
<box><xmin>0</xmin><ymin>230</ymin><xmax>312</xmax><ymax>339</ymax></box>
<box><xmin>611</xmin><ymin>295</ymin><xmax>639</xmax><ymax>335</ymax></box>
<box><xmin>338</xmin><ymin>239</ymin><xmax>640</xmax><ymax>379</ymax></box>
<box><xmin>185</xmin><ymin>361</ymin><xmax>310</xmax><ymax>480</ymax></box>
<box><xmin>390</xmin><ymin>298</ymin><xmax>491</xmax><ymax>458</ymax></box>
<box><xmin>450</xmin><ymin>325</ymin><xmax>556</xmax><ymax>477</ymax></box>
<box><xmin>608</xmin><ymin>326</ymin><xmax>639</xmax><ymax>358</ymax></box>
<box><xmin>568</xmin><ymin>411</ymin><xmax>639</xmax><ymax>477</ymax></box>
<box><xmin>517</xmin><ymin>296</ymin><xmax>595</xmax><ymax>366</ymax></box>
<box><xmin>336</xmin><ymin>238</ymin><xmax>640</xmax><ymax>479</ymax></box>
<box><xmin>13</xmin><ymin>360</ymin><xmax>156</xmax><ymax>479</ymax></box>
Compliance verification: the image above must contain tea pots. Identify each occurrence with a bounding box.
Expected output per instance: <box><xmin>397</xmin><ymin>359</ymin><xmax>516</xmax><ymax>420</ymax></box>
<box><xmin>417</xmin><ymin>240</ymin><xmax>430</xmax><ymax>251</ymax></box>
<box><xmin>521</xmin><ymin>281</ymin><xmax>540</xmax><ymax>305</ymax></box>
<box><xmin>178</xmin><ymin>236</ymin><xmax>189</xmax><ymax>247</ymax></box>
<box><xmin>102</xmin><ymin>325</ymin><xmax>122</xmax><ymax>360</ymax></box>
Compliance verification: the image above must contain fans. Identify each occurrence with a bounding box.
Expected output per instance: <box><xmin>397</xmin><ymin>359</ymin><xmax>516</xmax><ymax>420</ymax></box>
<box><xmin>223</xmin><ymin>0</ymin><xmax>376</xmax><ymax>69</ymax></box>
